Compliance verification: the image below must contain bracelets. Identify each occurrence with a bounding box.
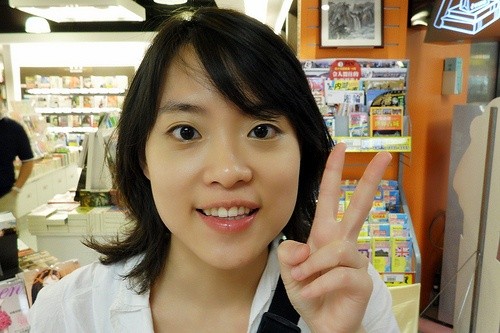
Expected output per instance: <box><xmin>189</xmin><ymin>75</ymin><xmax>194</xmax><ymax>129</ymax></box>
<box><xmin>12</xmin><ymin>187</ymin><xmax>20</xmax><ymax>192</ymax></box>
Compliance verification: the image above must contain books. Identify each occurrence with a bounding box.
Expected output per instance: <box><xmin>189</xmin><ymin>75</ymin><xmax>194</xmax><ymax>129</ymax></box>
<box><xmin>9</xmin><ymin>75</ymin><xmax>128</xmax><ymax>178</ymax></box>
<box><xmin>337</xmin><ymin>179</ymin><xmax>416</xmax><ymax>287</ymax></box>
<box><xmin>301</xmin><ymin>59</ymin><xmax>409</xmax><ymax>138</ymax></box>
<box><xmin>0</xmin><ymin>248</ymin><xmax>80</xmax><ymax>333</ymax></box>
<box><xmin>28</xmin><ymin>193</ymin><xmax>134</xmax><ymax>234</ymax></box>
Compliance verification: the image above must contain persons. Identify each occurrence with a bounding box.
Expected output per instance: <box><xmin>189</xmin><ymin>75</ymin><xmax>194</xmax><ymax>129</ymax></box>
<box><xmin>0</xmin><ymin>116</ymin><xmax>34</xmax><ymax>212</ymax></box>
<box><xmin>29</xmin><ymin>7</ymin><xmax>400</xmax><ymax>333</ymax></box>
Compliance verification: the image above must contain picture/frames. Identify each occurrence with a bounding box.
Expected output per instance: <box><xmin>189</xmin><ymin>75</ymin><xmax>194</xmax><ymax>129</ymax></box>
<box><xmin>319</xmin><ymin>0</ymin><xmax>384</xmax><ymax>48</ymax></box>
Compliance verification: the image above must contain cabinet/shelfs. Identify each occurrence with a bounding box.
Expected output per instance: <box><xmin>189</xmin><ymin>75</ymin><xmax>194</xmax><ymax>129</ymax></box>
<box><xmin>0</xmin><ymin>66</ymin><xmax>135</xmax><ymax>268</ymax></box>
<box><xmin>299</xmin><ymin>58</ymin><xmax>421</xmax><ymax>333</ymax></box>
